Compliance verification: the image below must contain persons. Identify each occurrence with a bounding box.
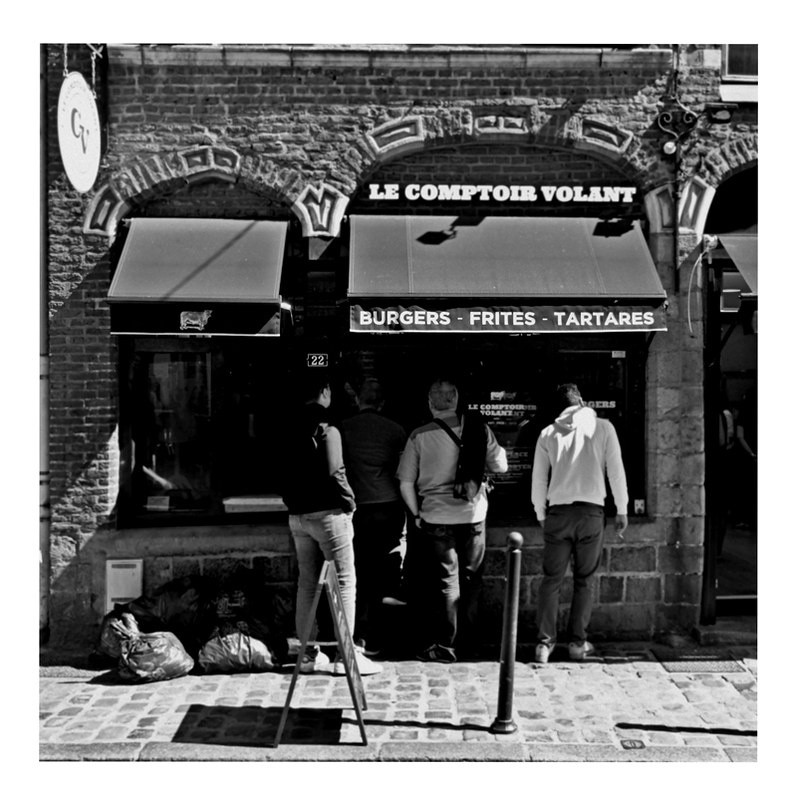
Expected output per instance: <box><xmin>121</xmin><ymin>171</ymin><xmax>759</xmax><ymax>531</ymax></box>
<box><xmin>530</xmin><ymin>384</ymin><xmax>629</xmax><ymax>663</ymax></box>
<box><xmin>394</xmin><ymin>381</ymin><xmax>509</xmax><ymax>667</ymax></box>
<box><xmin>334</xmin><ymin>380</ymin><xmax>408</xmax><ymax>658</ymax></box>
<box><xmin>281</xmin><ymin>379</ymin><xmax>380</xmax><ymax>677</ymax></box>
<box><xmin>735</xmin><ymin>389</ymin><xmax>757</xmax><ymax>529</ymax></box>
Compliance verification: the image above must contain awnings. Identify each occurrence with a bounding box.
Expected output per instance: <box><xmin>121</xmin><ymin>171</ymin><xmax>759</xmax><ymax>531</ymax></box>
<box><xmin>718</xmin><ymin>236</ymin><xmax>757</xmax><ymax>295</ymax></box>
<box><xmin>348</xmin><ymin>214</ymin><xmax>668</xmax><ymax>336</ymax></box>
<box><xmin>105</xmin><ymin>217</ymin><xmax>290</xmax><ymax>338</ymax></box>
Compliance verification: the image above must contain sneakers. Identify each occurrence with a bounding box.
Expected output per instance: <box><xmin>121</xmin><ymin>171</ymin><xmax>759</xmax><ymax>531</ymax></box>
<box><xmin>416</xmin><ymin>642</ymin><xmax>456</xmax><ymax>663</ymax></box>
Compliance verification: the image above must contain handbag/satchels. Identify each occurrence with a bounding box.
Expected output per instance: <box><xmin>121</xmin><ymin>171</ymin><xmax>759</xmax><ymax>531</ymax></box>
<box><xmin>482</xmin><ymin>472</ymin><xmax>495</xmax><ymax>494</ymax></box>
<box><xmin>198</xmin><ymin>627</ymin><xmax>274</xmax><ymax>674</ymax></box>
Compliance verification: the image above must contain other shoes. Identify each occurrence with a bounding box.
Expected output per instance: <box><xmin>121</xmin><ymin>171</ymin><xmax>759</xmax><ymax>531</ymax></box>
<box><xmin>334</xmin><ymin>651</ymin><xmax>378</xmax><ymax>675</ymax></box>
<box><xmin>535</xmin><ymin>642</ymin><xmax>556</xmax><ymax>664</ymax></box>
<box><xmin>300</xmin><ymin>645</ymin><xmax>334</xmax><ymax>673</ymax></box>
<box><xmin>568</xmin><ymin>638</ymin><xmax>595</xmax><ymax>660</ymax></box>
<box><xmin>353</xmin><ymin>644</ymin><xmax>379</xmax><ymax>655</ymax></box>
